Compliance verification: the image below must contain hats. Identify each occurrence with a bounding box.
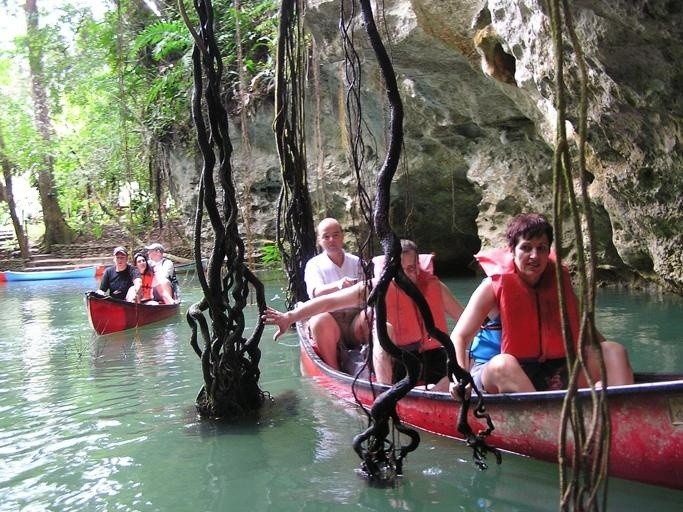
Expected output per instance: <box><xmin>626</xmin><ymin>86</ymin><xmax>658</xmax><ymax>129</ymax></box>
<box><xmin>114</xmin><ymin>246</ymin><xmax>128</xmax><ymax>256</ymax></box>
<box><xmin>146</xmin><ymin>243</ymin><xmax>164</xmax><ymax>254</ymax></box>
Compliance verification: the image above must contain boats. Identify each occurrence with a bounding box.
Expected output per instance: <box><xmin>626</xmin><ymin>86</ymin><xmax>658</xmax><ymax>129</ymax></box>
<box><xmin>83</xmin><ymin>291</ymin><xmax>184</xmax><ymax>336</ymax></box>
<box><xmin>0</xmin><ymin>275</ymin><xmax>106</xmax><ymax>293</ymax></box>
<box><xmin>287</xmin><ymin>296</ymin><xmax>682</xmax><ymax>495</ymax></box>
<box><xmin>0</xmin><ymin>264</ymin><xmax>108</xmax><ymax>281</ymax></box>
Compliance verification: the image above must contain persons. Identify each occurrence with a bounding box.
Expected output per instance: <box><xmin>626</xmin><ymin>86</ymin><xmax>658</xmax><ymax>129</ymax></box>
<box><xmin>144</xmin><ymin>243</ymin><xmax>177</xmax><ymax>304</ymax></box>
<box><xmin>302</xmin><ymin>216</ymin><xmax>369</xmax><ymax>372</ymax></box>
<box><xmin>260</xmin><ymin>238</ymin><xmax>468</xmax><ymax>392</ymax></box>
<box><xmin>94</xmin><ymin>245</ymin><xmax>143</xmax><ymax>305</ymax></box>
<box><xmin>132</xmin><ymin>252</ymin><xmax>159</xmax><ymax>306</ymax></box>
<box><xmin>445</xmin><ymin>213</ymin><xmax>635</xmax><ymax>403</ymax></box>
